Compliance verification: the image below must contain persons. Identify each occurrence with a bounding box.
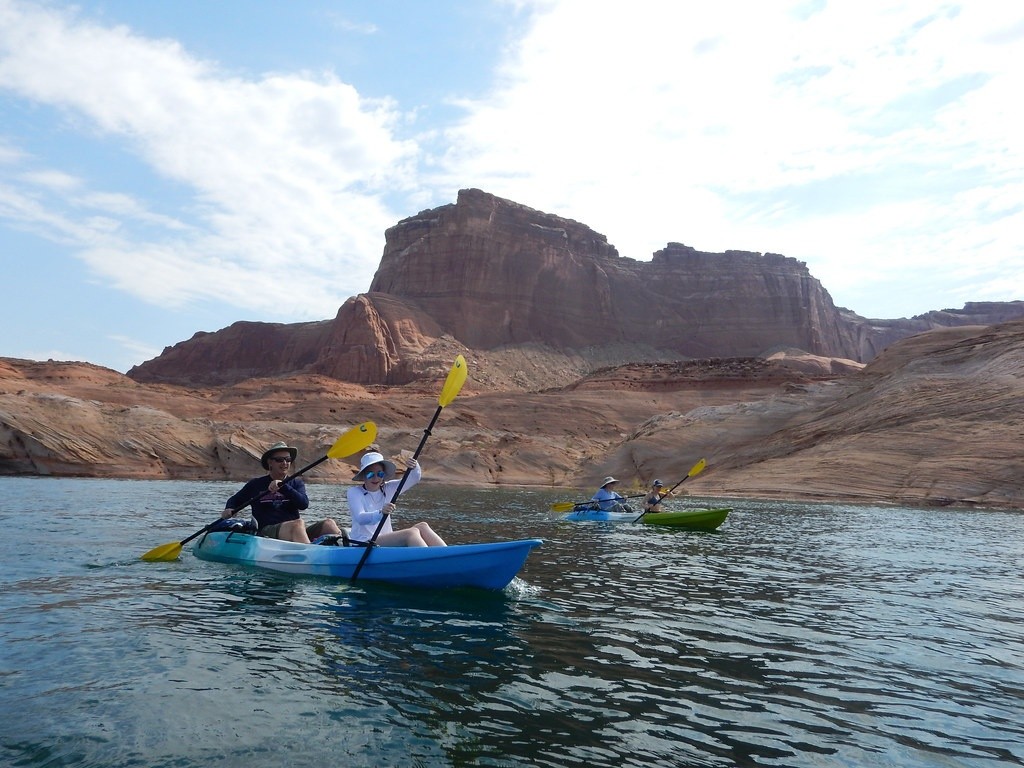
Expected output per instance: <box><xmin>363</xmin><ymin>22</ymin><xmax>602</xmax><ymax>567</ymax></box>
<box><xmin>221</xmin><ymin>441</ymin><xmax>342</xmax><ymax>544</ymax></box>
<box><xmin>641</xmin><ymin>479</ymin><xmax>675</xmax><ymax>512</ymax></box>
<box><xmin>346</xmin><ymin>453</ymin><xmax>448</xmax><ymax>547</ymax></box>
<box><xmin>589</xmin><ymin>476</ymin><xmax>633</xmax><ymax>513</ymax></box>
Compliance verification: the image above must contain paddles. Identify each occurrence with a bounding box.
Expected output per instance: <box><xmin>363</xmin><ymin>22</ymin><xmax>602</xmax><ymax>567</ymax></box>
<box><xmin>138</xmin><ymin>421</ymin><xmax>378</xmax><ymax>561</ymax></box>
<box><xmin>551</xmin><ymin>488</ymin><xmax>672</xmax><ymax>511</ymax></box>
<box><xmin>347</xmin><ymin>354</ymin><xmax>468</xmax><ymax>587</ymax></box>
<box><xmin>631</xmin><ymin>458</ymin><xmax>706</xmax><ymax>525</ymax></box>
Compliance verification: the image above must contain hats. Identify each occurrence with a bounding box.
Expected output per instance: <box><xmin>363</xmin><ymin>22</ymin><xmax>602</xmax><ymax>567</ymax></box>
<box><xmin>600</xmin><ymin>476</ymin><xmax>620</xmax><ymax>489</ymax></box>
<box><xmin>261</xmin><ymin>441</ymin><xmax>298</xmax><ymax>470</ymax></box>
<box><xmin>352</xmin><ymin>452</ymin><xmax>395</xmax><ymax>481</ymax></box>
<box><xmin>653</xmin><ymin>479</ymin><xmax>663</xmax><ymax>486</ymax></box>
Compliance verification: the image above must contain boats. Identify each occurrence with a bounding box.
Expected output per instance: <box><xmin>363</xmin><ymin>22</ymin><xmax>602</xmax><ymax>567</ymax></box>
<box><xmin>564</xmin><ymin>508</ymin><xmax>732</xmax><ymax>532</ymax></box>
<box><xmin>193</xmin><ymin>528</ymin><xmax>543</xmax><ymax>592</ymax></box>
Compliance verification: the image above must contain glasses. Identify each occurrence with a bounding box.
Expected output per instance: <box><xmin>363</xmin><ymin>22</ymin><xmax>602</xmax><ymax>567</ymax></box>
<box><xmin>363</xmin><ymin>471</ymin><xmax>386</xmax><ymax>479</ymax></box>
<box><xmin>270</xmin><ymin>456</ymin><xmax>291</xmax><ymax>463</ymax></box>
